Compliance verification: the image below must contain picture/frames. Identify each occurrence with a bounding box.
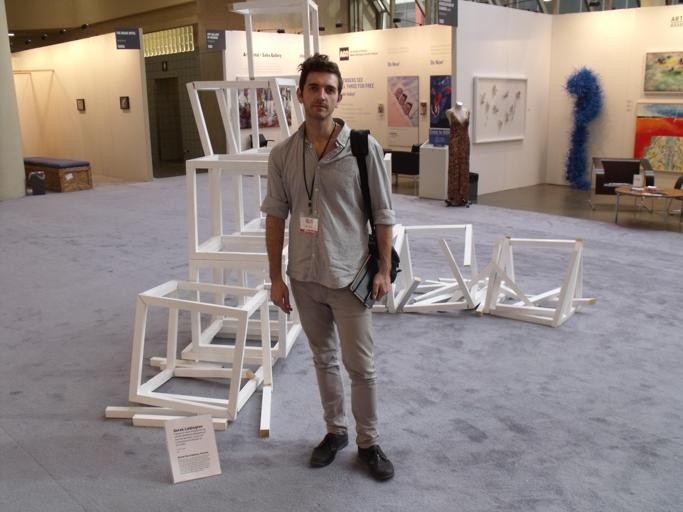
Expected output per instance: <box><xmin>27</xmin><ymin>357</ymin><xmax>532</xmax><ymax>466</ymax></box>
<box><xmin>120</xmin><ymin>96</ymin><xmax>129</xmax><ymax>109</ymax></box>
<box><xmin>472</xmin><ymin>75</ymin><xmax>528</xmax><ymax>143</ymax></box>
<box><xmin>77</xmin><ymin>99</ymin><xmax>85</xmax><ymax>111</ymax></box>
<box><xmin>642</xmin><ymin>50</ymin><xmax>683</xmax><ymax>94</ymax></box>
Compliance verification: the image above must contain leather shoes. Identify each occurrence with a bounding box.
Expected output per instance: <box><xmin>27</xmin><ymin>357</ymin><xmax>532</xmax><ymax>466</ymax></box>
<box><xmin>358</xmin><ymin>445</ymin><xmax>394</xmax><ymax>480</ymax></box>
<box><xmin>311</xmin><ymin>431</ymin><xmax>348</xmax><ymax>467</ymax></box>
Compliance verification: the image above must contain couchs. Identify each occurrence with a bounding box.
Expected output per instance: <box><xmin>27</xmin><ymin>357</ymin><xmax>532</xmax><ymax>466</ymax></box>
<box><xmin>588</xmin><ymin>156</ymin><xmax>655</xmax><ymax>212</ymax></box>
<box><xmin>383</xmin><ymin>143</ymin><xmax>419</xmax><ymax>185</ymax></box>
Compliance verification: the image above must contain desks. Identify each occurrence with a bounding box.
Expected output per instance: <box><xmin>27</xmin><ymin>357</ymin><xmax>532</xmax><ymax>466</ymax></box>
<box><xmin>419</xmin><ymin>139</ymin><xmax>449</xmax><ymax>200</ymax></box>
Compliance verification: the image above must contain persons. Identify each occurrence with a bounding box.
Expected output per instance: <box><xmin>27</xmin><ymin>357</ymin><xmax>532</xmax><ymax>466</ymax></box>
<box><xmin>260</xmin><ymin>54</ymin><xmax>402</xmax><ymax>481</ymax></box>
<box><xmin>445</xmin><ymin>102</ymin><xmax>470</xmax><ymax>206</ymax></box>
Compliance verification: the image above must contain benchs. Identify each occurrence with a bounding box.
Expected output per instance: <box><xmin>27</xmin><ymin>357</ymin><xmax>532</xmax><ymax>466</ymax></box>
<box><xmin>24</xmin><ymin>156</ymin><xmax>93</xmax><ymax>193</ymax></box>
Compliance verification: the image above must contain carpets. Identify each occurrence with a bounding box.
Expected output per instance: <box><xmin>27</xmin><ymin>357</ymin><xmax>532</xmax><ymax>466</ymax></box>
<box><xmin>1</xmin><ymin>173</ymin><xmax>683</xmax><ymax>511</ymax></box>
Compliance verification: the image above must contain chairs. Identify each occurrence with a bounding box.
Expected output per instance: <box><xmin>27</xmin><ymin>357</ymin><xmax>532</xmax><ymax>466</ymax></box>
<box><xmin>666</xmin><ymin>176</ymin><xmax>683</xmax><ymax>215</ymax></box>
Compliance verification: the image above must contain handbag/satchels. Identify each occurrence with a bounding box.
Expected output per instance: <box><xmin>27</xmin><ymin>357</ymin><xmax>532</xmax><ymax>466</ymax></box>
<box><xmin>369</xmin><ymin>235</ymin><xmax>400</xmax><ymax>283</ymax></box>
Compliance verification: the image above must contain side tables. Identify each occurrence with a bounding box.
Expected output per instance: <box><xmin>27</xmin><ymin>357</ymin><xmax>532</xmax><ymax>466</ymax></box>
<box><xmin>616</xmin><ymin>185</ymin><xmax>683</xmax><ymax>222</ymax></box>
